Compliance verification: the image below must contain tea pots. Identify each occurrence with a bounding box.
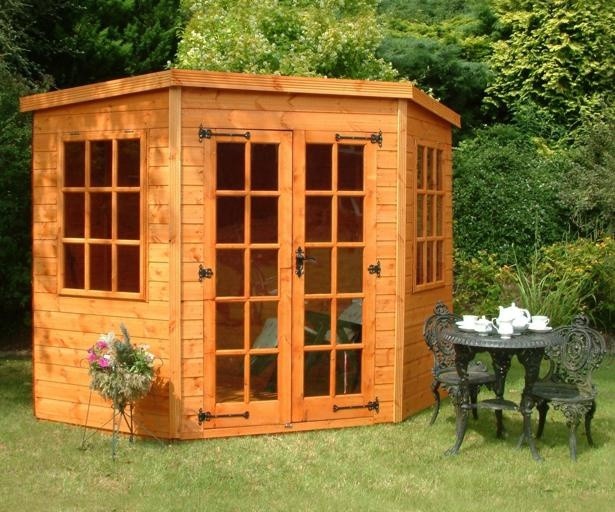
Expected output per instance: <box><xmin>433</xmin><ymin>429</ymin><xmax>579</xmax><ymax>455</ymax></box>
<box><xmin>491</xmin><ymin>315</ymin><xmax>516</xmax><ymax>339</ymax></box>
<box><xmin>475</xmin><ymin>315</ymin><xmax>493</xmax><ymax>337</ymax></box>
<box><xmin>496</xmin><ymin>302</ymin><xmax>531</xmax><ymax>336</ymax></box>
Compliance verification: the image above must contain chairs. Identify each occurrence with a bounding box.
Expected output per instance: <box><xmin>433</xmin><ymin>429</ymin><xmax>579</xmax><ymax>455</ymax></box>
<box><xmin>244</xmin><ymin>295</ymin><xmax>361</xmax><ymax>402</ymax></box>
<box><xmin>425</xmin><ymin>298</ymin><xmax>606</xmax><ymax>462</ymax></box>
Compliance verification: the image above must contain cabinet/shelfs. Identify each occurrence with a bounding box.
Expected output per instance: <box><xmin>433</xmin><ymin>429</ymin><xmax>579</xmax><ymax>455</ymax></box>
<box><xmin>80</xmin><ymin>384</ymin><xmax>167</xmax><ymax>462</ymax></box>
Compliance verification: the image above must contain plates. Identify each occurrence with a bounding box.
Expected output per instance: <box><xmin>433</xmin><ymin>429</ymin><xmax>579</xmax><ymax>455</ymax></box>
<box><xmin>527</xmin><ymin>327</ymin><xmax>553</xmax><ymax>334</ymax></box>
<box><xmin>455</xmin><ymin>320</ymin><xmax>478</xmax><ymax>327</ymax></box>
<box><xmin>458</xmin><ymin>326</ymin><xmax>475</xmax><ymax>333</ymax></box>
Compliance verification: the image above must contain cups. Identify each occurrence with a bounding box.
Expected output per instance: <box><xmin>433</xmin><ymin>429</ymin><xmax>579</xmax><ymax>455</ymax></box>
<box><xmin>531</xmin><ymin>316</ymin><xmax>550</xmax><ymax>328</ymax></box>
<box><xmin>462</xmin><ymin>314</ymin><xmax>480</xmax><ymax>325</ymax></box>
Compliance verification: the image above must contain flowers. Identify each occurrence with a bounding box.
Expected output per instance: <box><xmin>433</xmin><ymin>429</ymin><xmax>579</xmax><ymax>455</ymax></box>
<box><xmin>87</xmin><ymin>323</ymin><xmax>162</xmax><ymax>412</ymax></box>
